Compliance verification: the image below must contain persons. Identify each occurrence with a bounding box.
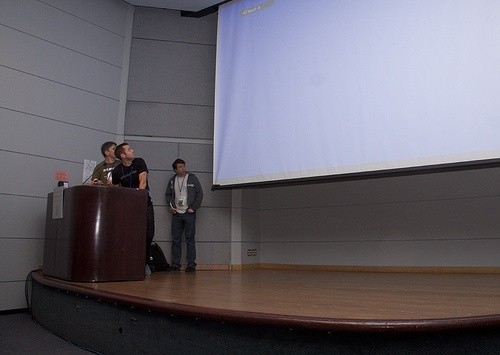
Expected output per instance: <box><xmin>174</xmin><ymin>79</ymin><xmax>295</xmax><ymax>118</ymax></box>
<box><xmin>92</xmin><ymin>142</ymin><xmax>155</xmax><ymax>276</ymax></box>
<box><xmin>91</xmin><ymin>141</ymin><xmax>121</xmax><ymax>186</ymax></box>
<box><xmin>165</xmin><ymin>158</ymin><xmax>204</xmax><ymax>272</ymax></box>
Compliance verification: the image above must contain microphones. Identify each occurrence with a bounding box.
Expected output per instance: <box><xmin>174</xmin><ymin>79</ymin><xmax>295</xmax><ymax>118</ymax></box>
<box><xmin>82</xmin><ymin>163</ymin><xmax>108</xmax><ymax>184</ymax></box>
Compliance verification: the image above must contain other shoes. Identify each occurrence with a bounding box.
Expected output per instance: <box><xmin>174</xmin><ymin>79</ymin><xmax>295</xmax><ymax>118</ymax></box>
<box><xmin>185</xmin><ymin>266</ymin><xmax>195</xmax><ymax>272</ymax></box>
<box><xmin>170</xmin><ymin>266</ymin><xmax>180</xmax><ymax>271</ymax></box>
<box><xmin>145</xmin><ymin>264</ymin><xmax>152</xmax><ymax>276</ymax></box>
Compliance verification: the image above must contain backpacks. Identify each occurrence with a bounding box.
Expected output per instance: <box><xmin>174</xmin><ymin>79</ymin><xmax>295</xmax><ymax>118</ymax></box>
<box><xmin>147</xmin><ymin>241</ymin><xmax>170</xmax><ymax>272</ymax></box>
